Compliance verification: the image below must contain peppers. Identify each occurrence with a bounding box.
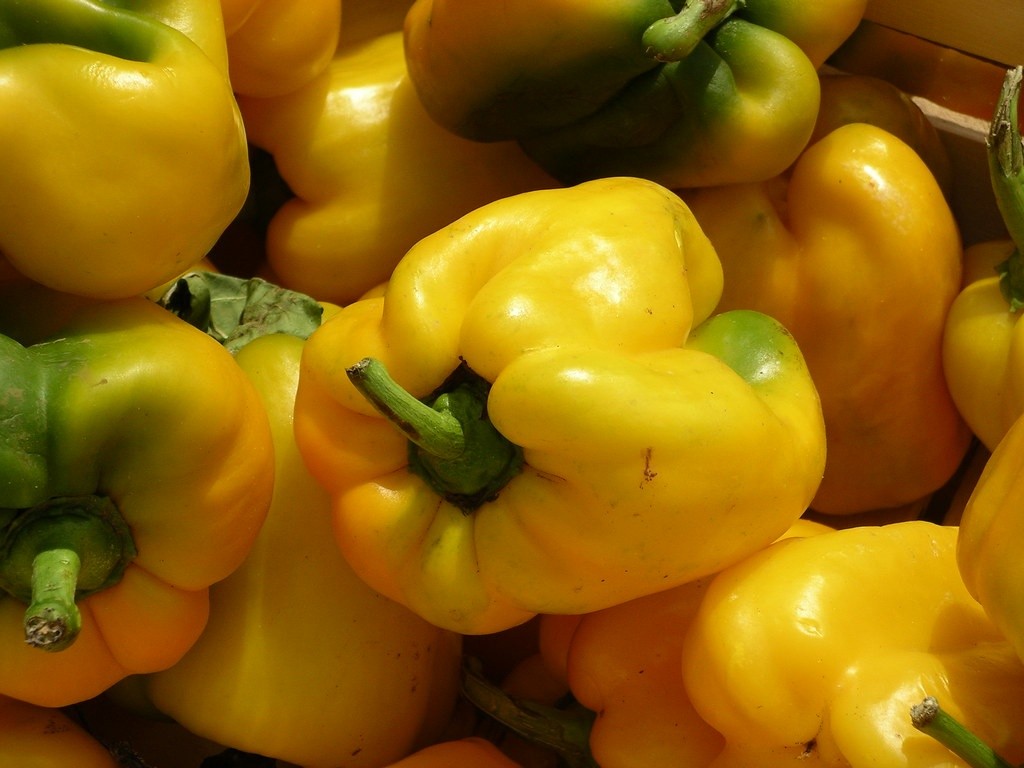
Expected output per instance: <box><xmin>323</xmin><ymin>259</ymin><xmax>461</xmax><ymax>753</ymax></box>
<box><xmin>0</xmin><ymin>0</ymin><xmax>1024</xmax><ymax>768</ymax></box>
